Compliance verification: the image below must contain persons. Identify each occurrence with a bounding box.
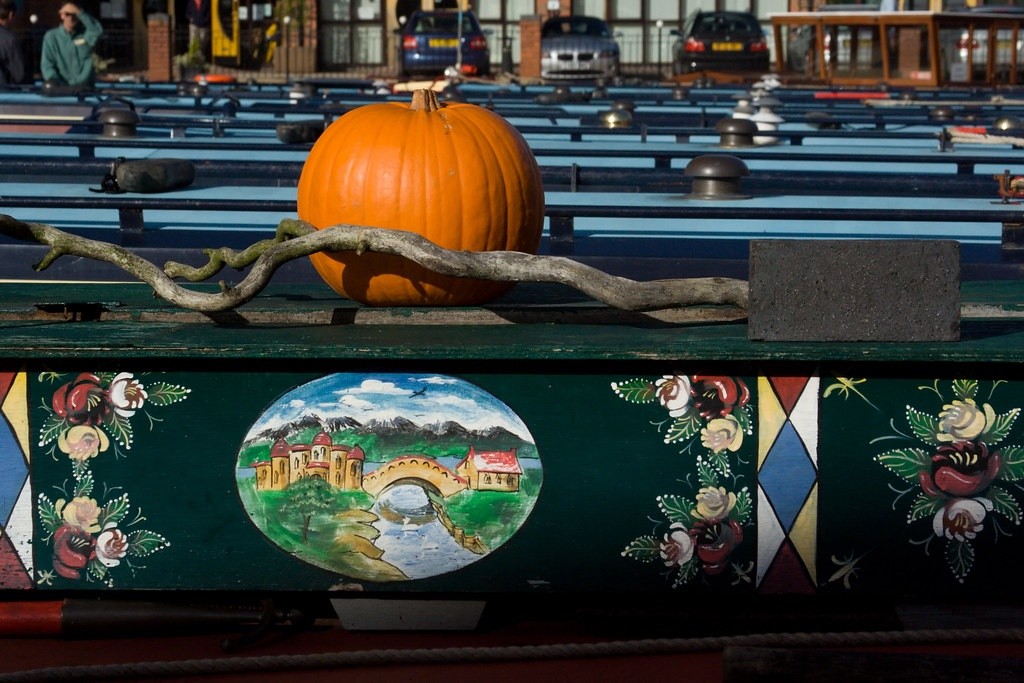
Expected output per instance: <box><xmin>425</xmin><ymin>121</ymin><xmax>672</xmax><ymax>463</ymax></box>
<box><xmin>42</xmin><ymin>2</ymin><xmax>103</xmax><ymax>93</ymax></box>
<box><xmin>0</xmin><ymin>0</ymin><xmax>27</xmax><ymax>88</ymax></box>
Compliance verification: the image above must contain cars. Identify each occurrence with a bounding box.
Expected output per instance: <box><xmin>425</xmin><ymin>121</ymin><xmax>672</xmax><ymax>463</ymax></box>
<box><xmin>669</xmin><ymin>9</ymin><xmax>770</xmax><ymax>78</ymax></box>
<box><xmin>540</xmin><ymin>11</ymin><xmax>624</xmax><ymax>84</ymax></box>
<box><xmin>393</xmin><ymin>8</ymin><xmax>493</xmax><ymax>79</ymax></box>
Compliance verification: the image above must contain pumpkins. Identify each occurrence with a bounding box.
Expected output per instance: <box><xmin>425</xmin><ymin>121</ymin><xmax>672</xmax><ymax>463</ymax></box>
<box><xmin>296</xmin><ymin>88</ymin><xmax>544</xmax><ymax>307</ymax></box>
